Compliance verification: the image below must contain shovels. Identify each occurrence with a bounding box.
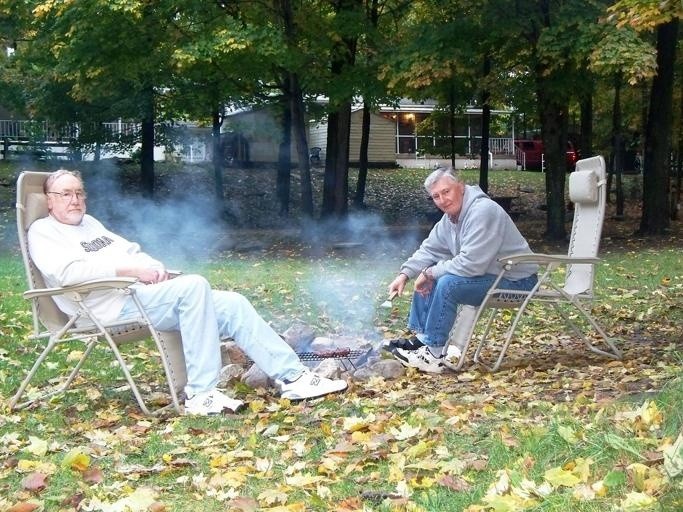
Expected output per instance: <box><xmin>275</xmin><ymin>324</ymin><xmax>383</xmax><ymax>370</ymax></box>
<box><xmin>380</xmin><ymin>289</ymin><xmax>399</xmax><ymax>308</ymax></box>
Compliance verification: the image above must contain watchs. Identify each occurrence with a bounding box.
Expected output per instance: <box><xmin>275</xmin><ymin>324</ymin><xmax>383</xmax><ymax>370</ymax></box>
<box><xmin>421</xmin><ymin>266</ymin><xmax>429</xmax><ymax>281</ymax></box>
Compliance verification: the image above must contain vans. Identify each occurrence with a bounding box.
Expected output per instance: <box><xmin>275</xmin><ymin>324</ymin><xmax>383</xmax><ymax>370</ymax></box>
<box><xmin>513</xmin><ymin>138</ymin><xmax>577</xmax><ymax>168</ymax></box>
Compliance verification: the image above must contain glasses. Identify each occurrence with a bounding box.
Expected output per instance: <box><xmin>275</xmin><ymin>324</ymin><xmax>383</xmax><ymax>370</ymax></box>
<box><xmin>45</xmin><ymin>189</ymin><xmax>85</xmax><ymax>201</ymax></box>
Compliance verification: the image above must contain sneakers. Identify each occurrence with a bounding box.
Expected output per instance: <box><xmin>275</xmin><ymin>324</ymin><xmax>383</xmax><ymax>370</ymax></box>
<box><xmin>183</xmin><ymin>388</ymin><xmax>247</xmax><ymax>414</ymax></box>
<box><xmin>275</xmin><ymin>370</ymin><xmax>348</xmax><ymax>400</ymax></box>
<box><xmin>380</xmin><ymin>333</ymin><xmax>423</xmax><ymax>356</ymax></box>
<box><xmin>391</xmin><ymin>348</ymin><xmax>443</xmax><ymax>373</ymax></box>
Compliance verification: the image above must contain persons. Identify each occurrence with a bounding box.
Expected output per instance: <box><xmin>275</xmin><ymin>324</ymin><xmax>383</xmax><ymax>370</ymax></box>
<box><xmin>388</xmin><ymin>168</ymin><xmax>538</xmax><ymax>374</ymax></box>
<box><xmin>27</xmin><ymin>169</ymin><xmax>348</xmax><ymax>414</ymax></box>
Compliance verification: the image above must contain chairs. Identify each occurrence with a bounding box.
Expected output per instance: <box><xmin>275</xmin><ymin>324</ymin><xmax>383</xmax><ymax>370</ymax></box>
<box><xmin>6</xmin><ymin>171</ymin><xmax>186</xmax><ymax>419</ymax></box>
<box><xmin>438</xmin><ymin>154</ymin><xmax>623</xmax><ymax>374</ymax></box>
